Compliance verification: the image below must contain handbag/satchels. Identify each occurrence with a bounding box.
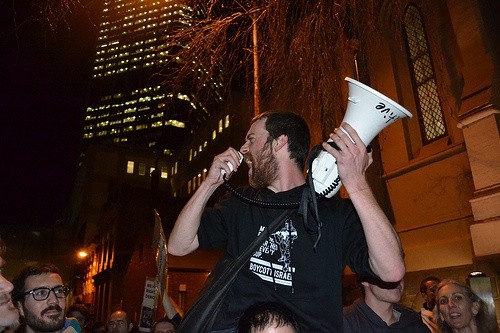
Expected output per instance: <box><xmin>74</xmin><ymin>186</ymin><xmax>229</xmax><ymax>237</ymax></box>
<box><xmin>176</xmin><ymin>254</ymin><xmax>237</xmax><ymax>333</ymax></box>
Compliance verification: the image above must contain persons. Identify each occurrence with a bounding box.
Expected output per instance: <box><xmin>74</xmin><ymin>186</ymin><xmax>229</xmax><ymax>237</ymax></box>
<box><xmin>340</xmin><ymin>276</ymin><xmax>500</xmax><ymax>333</ymax></box>
<box><xmin>167</xmin><ymin>111</ymin><xmax>404</xmax><ymax>333</ymax></box>
<box><xmin>0</xmin><ymin>252</ymin><xmax>187</xmax><ymax>333</ymax></box>
<box><xmin>237</xmin><ymin>300</ymin><xmax>304</xmax><ymax>333</ymax></box>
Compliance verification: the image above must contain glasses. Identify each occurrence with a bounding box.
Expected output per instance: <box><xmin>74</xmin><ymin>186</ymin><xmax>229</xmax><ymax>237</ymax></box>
<box><xmin>20</xmin><ymin>286</ymin><xmax>70</xmax><ymax>301</ymax></box>
<box><xmin>108</xmin><ymin>321</ymin><xmax>129</xmax><ymax>328</ymax></box>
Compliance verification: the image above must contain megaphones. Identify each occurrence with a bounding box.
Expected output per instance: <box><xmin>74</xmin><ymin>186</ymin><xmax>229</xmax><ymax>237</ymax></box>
<box><xmin>306</xmin><ymin>75</ymin><xmax>414</xmax><ymax>200</ymax></box>
